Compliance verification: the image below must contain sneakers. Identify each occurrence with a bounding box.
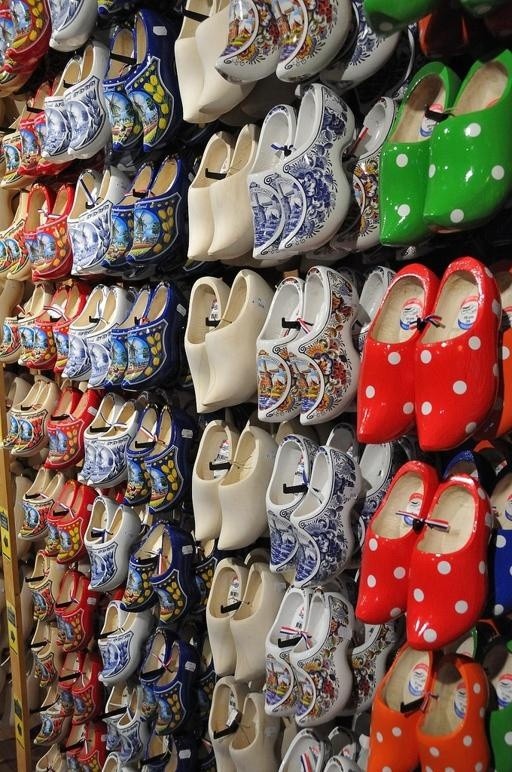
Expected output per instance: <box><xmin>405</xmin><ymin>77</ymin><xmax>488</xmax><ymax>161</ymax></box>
<box><xmin>84</xmin><ymin>495</ymin><xmax>142</xmax><ymax>592</ymax></box>
<box><xmin>377</xmin><ymin>61</ymin><xmax>461</xmax><ymax>248</ymax></box>
<box><xmin>67</xmin><ymin>721</ymin><xmax>92</xmax><ymax>771</ymax></box>
<box><xmin>139</xmin><ymin>627</ymin><xmax>175</xmax><ymax>722</ymax></box>
<box><xmin>57</xmin><ymin>484</ymin><xmax>100</xmax><ymax>564</ymax></box>
<box><xmin>246</xmin><ymin>105</ymin><xmax>298</xmax><ymax>260</ymax></box>
<box><xmin>1</xmin><ymin>280</ymin><xmax>92</xmax><ymax>377</ymax></box>
<box><xmin>0</xmin><ymin>183</ymin><xmax>75</xmax><ymax>281</ymax></box>
<box><xmin>184</xmin><ymin>277</ymin><xmax>231</xmax><ymax>413</ymax></box>
<box><xmin>99</xmin><ymin>610</ymin><xmax>155</xmax><ymax>684</ymax></box>
<box><xmin>320</xmin><ymin>0</ymin><xmax>407</xmax><ymax>92</ymax></box>
<box><xmin>1</xmin><ymin>379</ymin><xmax>60</xmax><ymax>457</ymax></box>
<box><xmin>102</xmin><ymin>160</ymin><xmax>159</xmax><ymax>272</ymax></box>
<box><xmin>99</xmin><ymin>600</ymin><xmax>127</xmax><ymax>687</ymax></box>
<box><xmin>42</xmin><ymin>54</ymin><xmax>83</xmax><ymax>164</ymax></box>
<box><xmin>195</xmin><ymin>1</ymin><xmax>256</xmax><ymax>116</ymax></box>
<box><xmin>413</xmin><ymin>653</ymin><xmax>492</xmax><ymax>770</ymax></box>
<box><xmin>365</xmin><ymin>646</ymin><xmax>438</xmax><ymax>771</ymax></box>
<box><xmin>38</xmin><ymin>622</ymin><xmax>64</xmax><ymax>687</ymax></box>
<box><xmin>256</xmin><ymin>275</ymin><xmax>304</xmax><ymax>422</ymax></box>
<box><xmin>124</xmin><ymin>401</ymin><xmax>162</xmax><ymax>506</ymax></box>
<box><xmin>154</xmin><ymin>641</ymin><xmax>201</xmax><ymax>737</ymax></box>
<box><xmin>415</xmin><ymin>255</ymin><xmax>502</xmax><ymax>454</ymax></box>
<box><xmin>290</xmin><ymin>586</ymin><xmax>354</xmax><ymax>727</ymax></box>
<box><xmin>18</xmin><ymin>467</ymin><xmax>66</xmax><ymax>541</ymax></box>
<box><xmin>406</xmin><ymin>472</ymin><xmax>493</xmax><ymax>649</ymax></box>
<box><xmin>229</xmin><ymin>693</ymin><xmax>281</xmax><ymax>771</ymax></box>
<box><xmin>44</xmin><ymin>480</ymin><xmax>83</xmax><ymax>557</ymax></box>
<box><xmin>277</xmin><ymin>714</ymin><xmax>365</xmax><ymax>772</ymax></box>
<box><xmin>34</xmin><ymin>686</ymin><xmax>72</xmax><ymax>746</ymax></box>
<box><xmin>102</xmin><ymin>23</ymin><xmax>143</xmax><ymax>152</ymax></box>
<box><xmin>78</xmin><ymin>721</ymin><xmax>107</xmax><ymax>771</ymax></box>
<box><xmin>339</xmin><ymin>621</ymin><xmax>405</xmax><ymax>716</ymax></box>
<box><xmin>265</xmin><ymin>434</ymin><xmax>319</xmax><ymax>572</ymax></box>
<box><xmin>44</xmin><ymin>387</ymin><xmax>106</xmax><ymax>471</ymax></box>
<box><xmin>346</xmin><ymin>441</ymin><xmax>409</xmax><ymax>569</ymax></box>
<box><xmin>125</xmin><ymin>10</ymin><xmax>177</xmax><ymax>153</ymax></box>
<box><xmin>290</xmin><ymin>446</ymin><xmax>362</xmax><ymax>587</ymax></box>
<box><xmin>192</xmin><ymin>419</ymin><xmax>241</xmax><ymax>541</ymax></box>
<box><xmin>206</xmin><ymin>557</ymin><xmax>249</xmax><ymax>677</ymax></box>
<box><xmin>0</xmin><ymin>74</ymin><xmax>69</xmax><ymax>191</ymax></box>
<box><xmin>105</xmin><ymin>681</ymin><xmax>135</xmax><ymax>751</ymax></box>
<box><xmin>0</xmin><ymin>0</ymin><xmax>99</xmax><ymax>95</ymax></box>
<box><xmin>61</xmin><ymin>284</ymin><xmax>110</xmax><ymax>382</ymax></box>
<box><xmin>36</xmin><ymin>743</ymin><xmax>68</xmax><ymax>772</ymax></box>
<box><xmin>354</xmin><ymin>461</ymin><xmax>438</xmax><ymax>624</ymax></box>
<box><xmin>72</xmin><ymin>653</ymin><xmax>104</xmax><ymax>724</ymax></box>
<box><xmin>118</xmin><ymin>685</ymin><xmax>157</xmax><ymax>768</ymax></box>
<box><xmin>102</xmin><ymin>751</ymin><xmax>138</xmax><ymax>772</ymax></box>
<box><xmin>188</xmin><ymin>131</ymin><xmax>235</xmax><ymax>261</ymax></box>
<box><xmin>122</xmin><ymin>277</ymin><xmax>189</xmax><ymax>393</ymax></box>
<box><xmin>145</xmin><ymin>405</ymin><xmax>199</xmax><ymax>516</ymax></box>
<box><xmin>127</xmin><ymin>152</ymin><xmax>195</xmax><ymax>268</ymax></box>
<box><xmin>230</xmin><ymin>562</ymin><xmax>287</xmax><ymax>682</ymax></box>
<box><xmin>349</xmin><ymin>265</ymin><xmax>395</xmax><ymax>359</ymax></box>
<box><xmin>273</xmin><ymin>83</ymin><xmax>356</xmax><ymax>251</ymax></box>
<box><xmin>422</xmin><ymin>50</ymin><xmax>512</xmax><ymax>234</ymax></box>
<box><xmin>357</xmin><ymin>262</ymin><xmax>439</xmax><ymax>445</ymax></box>
<box><xmin>263</xmin><ymin>586</ymin><xmax>316</xmax><ymax>716</ymax></box>
<box><xmin>217</xmin><ymin>424</ymin><xmax>278</xmax><ymax>551</ymax></box>
<box><xmin>174</xmin><ymin>1</ymin><xmax>219</xmax><ymax>124</ymax></box>
<box><xmin>212</xmin><ymin>0</ymin><xmax>282</xmax><ymax>85</ymax></box>
<box><xmin>24</xmin><ymin>549</ymin><xmax>67</xmax><ymax>622</ymax></box>
<box><xmin>329</xmin><ymin>96</ymin><xmax>398</xmax><ymax>254</ymax></box>
<box><xmin>85</xmin><ymin>286</ymin><xmax>136</xmax><ymax>392</ymax></box>
<box><xmin>120</xmin><ymin>517</ymin><xmax>182</xmax><ymax>612</ymax></box>
<box><xmin>141</xmin><ymin>730</ymin><xmax>199</xmax><ymax>771</ymax></box>
<box><xmin>30</xmin><ymin>619</ymin><xmax>50</xmax><ymax>678</ymax></box>
<box><xmin>101</xmin><ymin>282</ymin><xmax>160</xmax><ymax>392</ymax></box>
<box><xmin>87</xmin><ymin>399</ymin><xmax>144</xmax><ymax>488</ymax></box>
<box><xmin>202</xmin><ymin>270</ymin><xmax>274</xmax><ymax>408</ymax></box>
<box><xmin>208</xmin><ymin>676</ymin><xmax>251</xmax><ymax>772</ymax></box>
<box><xmin>77</xmin><ymin>392</ymin><xmax>126</xmax><ymax>485</ymax></box>
<box><xmin>67</xmin><ymin>166</ymin><xmax>131</xmax><ymax>276</ymax></box>
<box><xmin>207</xmin><ymin>122</ymin><xmax>261</xmax><ymax>260</ymax></box>
<box><xmin>150</xmin><ymin>524</ymin><xmax>201</xmax><ymax>629</ymax></box>
<box><xmin>63</xmin><ymin>40</ymin><xmax>112</xmax><ymax>159</ymax></box>
<box><xmin>57</xmin><ymin>652</ymin><xmax>85</xmax><ymax>717</ymax></box>
<box><xmin>54</xmin><ymin>569</ymin><xmax>102</xmax><ymax>652</ymax></box>
<box><xmin>268</xmin><ymin>1</ymin><xmax>353</xmax><ymax>82</ymax></box>
<box><xmin>288</xmin><ymin>265</ymin><xmax>358</xmax><ymax>425</ymax></box>
